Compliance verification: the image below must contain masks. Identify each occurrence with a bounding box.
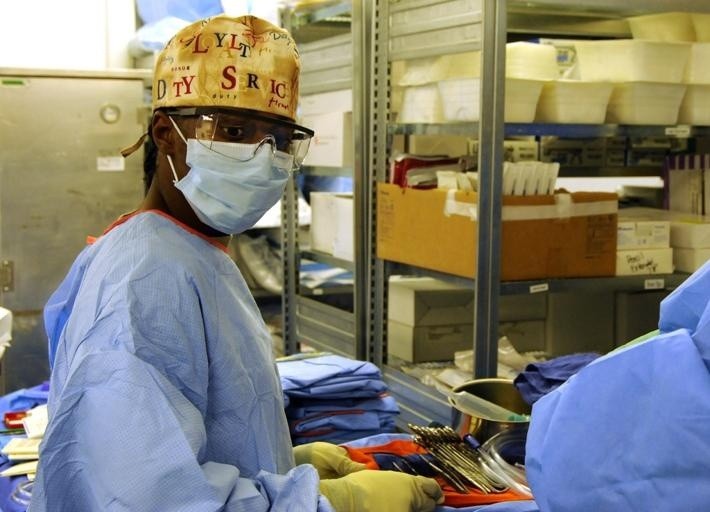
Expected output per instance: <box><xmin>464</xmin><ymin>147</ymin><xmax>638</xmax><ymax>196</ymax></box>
<box><xmin>162</xmin><ymin>114</ymin><xmax>298</xmax><ymax>235</ymax></box>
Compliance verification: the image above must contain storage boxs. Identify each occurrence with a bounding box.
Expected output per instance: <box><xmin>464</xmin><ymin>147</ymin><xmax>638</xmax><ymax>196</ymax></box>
<box><xmin>374</xmin><ymin>182</ymin><xmax>618</xmax><ymax>283</ymax></box>
<box><xmin>296</xmin><ymin>111</ymin><xmax>353</xmax><ymax>169</ymax></box>
<box><xmin>613</xmin><ymin>291</ymin><xmax>668</xmax><ymax>347</ymax></box>
<box><xmin>309</xmin><ymin>191</ymin><xmax>356</xmax><ymax>264</ymax></box>
<box><xmin>545</xmin><ymin>290</ymin><xmax>616</xmax><ymax>357</ymax></box>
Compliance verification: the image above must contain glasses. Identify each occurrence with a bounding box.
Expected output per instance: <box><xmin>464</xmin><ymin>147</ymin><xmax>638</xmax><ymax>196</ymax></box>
<box><xmin>171</xmin><ymin>106</ymin><xmax>314</xmax><ymax>171</ymax></box>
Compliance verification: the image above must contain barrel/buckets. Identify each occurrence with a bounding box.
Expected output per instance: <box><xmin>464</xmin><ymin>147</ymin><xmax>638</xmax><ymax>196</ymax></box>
<box><xmin>448</xmin><ymin>376</ymin><xmax>532</xmax><ymax>448</ymax></box>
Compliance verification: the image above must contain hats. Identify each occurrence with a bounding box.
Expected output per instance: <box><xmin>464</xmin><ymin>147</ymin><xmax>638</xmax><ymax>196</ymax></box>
<box><xmin>151</xmin><ymin>15</ymin><xmax>300</xmax><ymax>120</ymax></box>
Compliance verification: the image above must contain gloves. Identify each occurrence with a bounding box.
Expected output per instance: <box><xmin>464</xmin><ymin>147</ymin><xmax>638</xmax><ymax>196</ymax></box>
<box><xmin>294</xmin><ymin>440</ymin><xmax>364</xmax><ymax>479</ymax></box>
<box><xmin>319</xmin><ymin>470</ymin><xmax>446</xmax><ymax>511</ymax></box>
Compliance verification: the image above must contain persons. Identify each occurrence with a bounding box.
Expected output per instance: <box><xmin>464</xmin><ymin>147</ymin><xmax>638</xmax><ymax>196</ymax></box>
<box><xmin>31</xmin><ymin>12</ymin><xmax>451</xmax><ymax>510</ymax></box>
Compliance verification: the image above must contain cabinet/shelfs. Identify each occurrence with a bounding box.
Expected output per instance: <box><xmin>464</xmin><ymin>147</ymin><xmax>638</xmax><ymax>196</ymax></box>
<box><xmin>0</xmin><ymin>70</ymin><xmax>152</xmax><ymax>395</ymax></box>
<box><xmin>368</xmin><ymin>1</ymin><xmax>710</xmax><ymax>445</ymax></box>
<box><xmin>284</xmin><ymin>0</ymin><xmax>396</xmax><ymax>365</ymax></box>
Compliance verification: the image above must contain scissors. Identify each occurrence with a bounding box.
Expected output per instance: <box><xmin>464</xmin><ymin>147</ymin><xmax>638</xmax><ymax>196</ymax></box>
<box><xmin>407</xmin><ymin>424</ymin><xmax>507</xmax><ymax>495</ymax></box>
